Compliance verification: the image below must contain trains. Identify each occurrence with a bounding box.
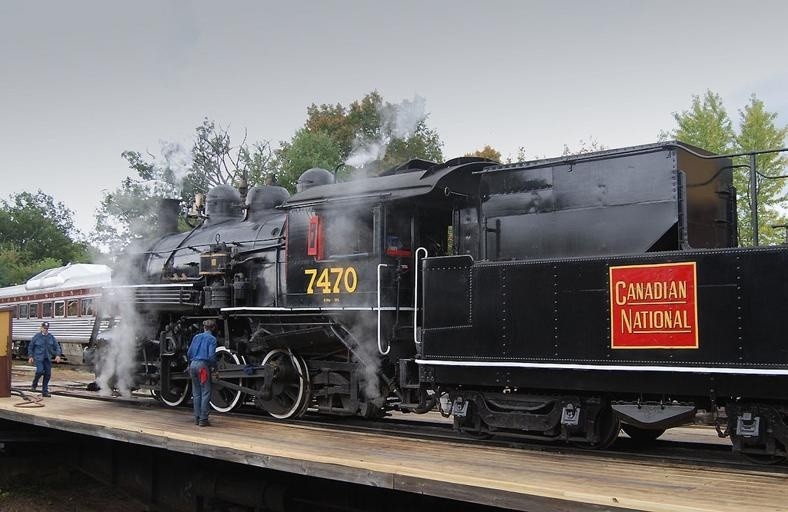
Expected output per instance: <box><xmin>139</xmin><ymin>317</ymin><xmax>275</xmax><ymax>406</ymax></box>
<box><xmin>83</xmin><ymin>138</ymin><xmax>787</xmax><ymax>467</ymax></box>
<box><xmin>0</xmin><ymin>262</ymin><xmax>122</xmax><ymax>360</ymax></box>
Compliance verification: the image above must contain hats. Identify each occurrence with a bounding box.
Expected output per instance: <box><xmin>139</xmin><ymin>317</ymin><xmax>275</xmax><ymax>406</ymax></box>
<box><xmin>42</xmin><ymin>322</ymin><xmax>49</xmax><ymax>328</ymax></box>
<box><xmin>203</xmin><ymin>319</ymin><xmax>216</xmax><ymax>327</ymax></box>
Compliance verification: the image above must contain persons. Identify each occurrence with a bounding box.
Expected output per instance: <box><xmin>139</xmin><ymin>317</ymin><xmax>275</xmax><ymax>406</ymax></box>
<box><xmin>27</xmin><ymin>322</ymin><xmax>62</xmax><ymax>397</ymax></box>
<box><xmin>187</xmin><ymin>320</ymin><xmax>218</xmax><ymax>427</ymax></box>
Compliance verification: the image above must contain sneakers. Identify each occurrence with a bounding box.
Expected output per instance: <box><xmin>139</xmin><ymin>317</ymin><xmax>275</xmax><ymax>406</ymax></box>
<box><xmin>194</xmin><ymin>419</ymin><xmax>199</xmax><ymax>425</ymax></box>
<box><xmin>32</xmin><ymin>384</ymin><xmax>36</xmax><ymax>391</ymax></box>
<box><xmin>42</xmin><ymin>392</ymin><xmax>51</xmax><ymax>397</ymax></box>
<box><xmin>199</xmin><ymin>420</ymin><xmax>212</xmax><ymax>426</ymax></box>
